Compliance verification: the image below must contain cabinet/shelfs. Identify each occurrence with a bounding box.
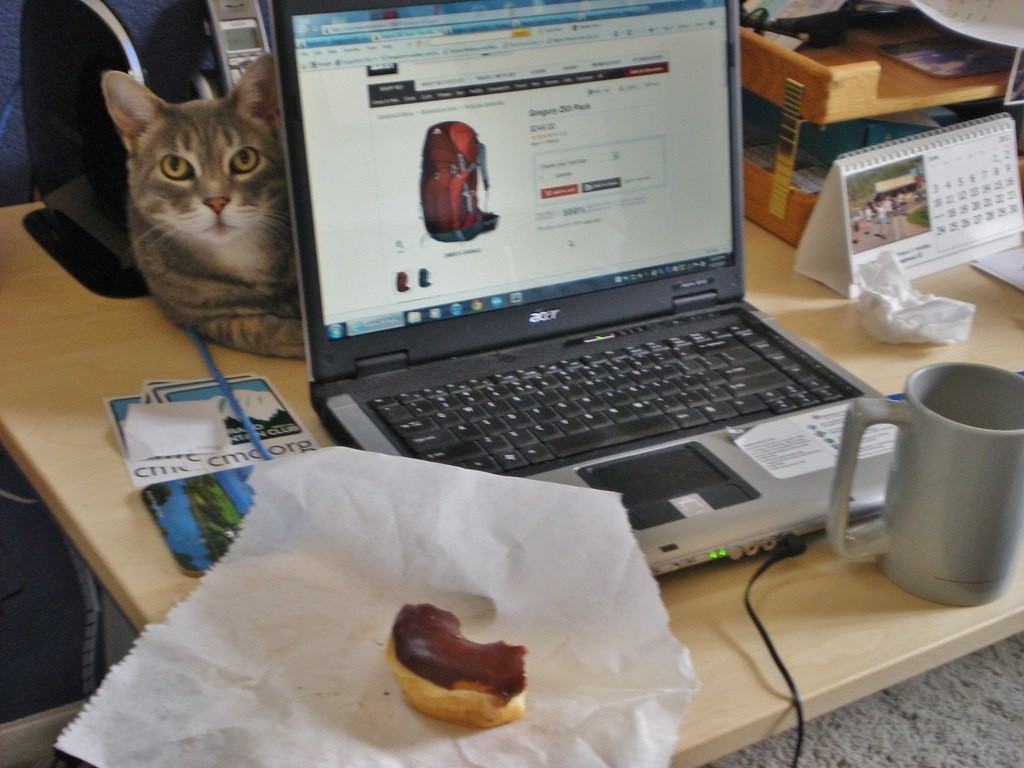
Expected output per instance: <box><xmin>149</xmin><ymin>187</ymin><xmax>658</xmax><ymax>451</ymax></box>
<box><xmin>737</xmin><ymin>0</ymin><xmax>1024</xmax><ymax>249</ymax></box>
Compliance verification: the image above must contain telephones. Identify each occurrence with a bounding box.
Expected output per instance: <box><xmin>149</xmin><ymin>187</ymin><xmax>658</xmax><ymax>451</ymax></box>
<box><xmin>195</xmin><ymin>0</ymin><xmax>271</xmax><ymax>107</ymax></box>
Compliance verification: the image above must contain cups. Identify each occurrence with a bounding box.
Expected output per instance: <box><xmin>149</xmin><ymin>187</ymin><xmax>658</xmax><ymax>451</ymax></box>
<box><xmin>824</xmin><ymin>363</ymin><xmax>1024</xmax><ymax>605</ymax></box>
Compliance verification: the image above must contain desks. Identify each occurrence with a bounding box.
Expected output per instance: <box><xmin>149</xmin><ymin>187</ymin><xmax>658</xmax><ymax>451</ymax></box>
<box><xmin>1</xmin><ymin>200</ymin><xmax>1023</xmax><ymax>768</ymax></box>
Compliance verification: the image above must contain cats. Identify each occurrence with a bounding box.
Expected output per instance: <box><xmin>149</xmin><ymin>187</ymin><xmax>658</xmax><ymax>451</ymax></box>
<box><xmin>99</xmin><ymin>51</ymin><xmax>305</xmax><ymax>364</ymax></box>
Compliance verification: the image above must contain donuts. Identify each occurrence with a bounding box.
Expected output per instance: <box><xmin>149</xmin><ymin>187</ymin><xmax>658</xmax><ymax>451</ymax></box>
<box><xmin>386</xmin><ymin>601</ymin><xmax>530</xmax><ymax>730</ymax></box>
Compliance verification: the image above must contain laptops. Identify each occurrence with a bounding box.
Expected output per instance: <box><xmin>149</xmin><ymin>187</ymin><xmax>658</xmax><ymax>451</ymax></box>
<box><xmin>268</xmin><ymin>0</ymin><xmax>906</xmax><ymax>577</ymax></box>
